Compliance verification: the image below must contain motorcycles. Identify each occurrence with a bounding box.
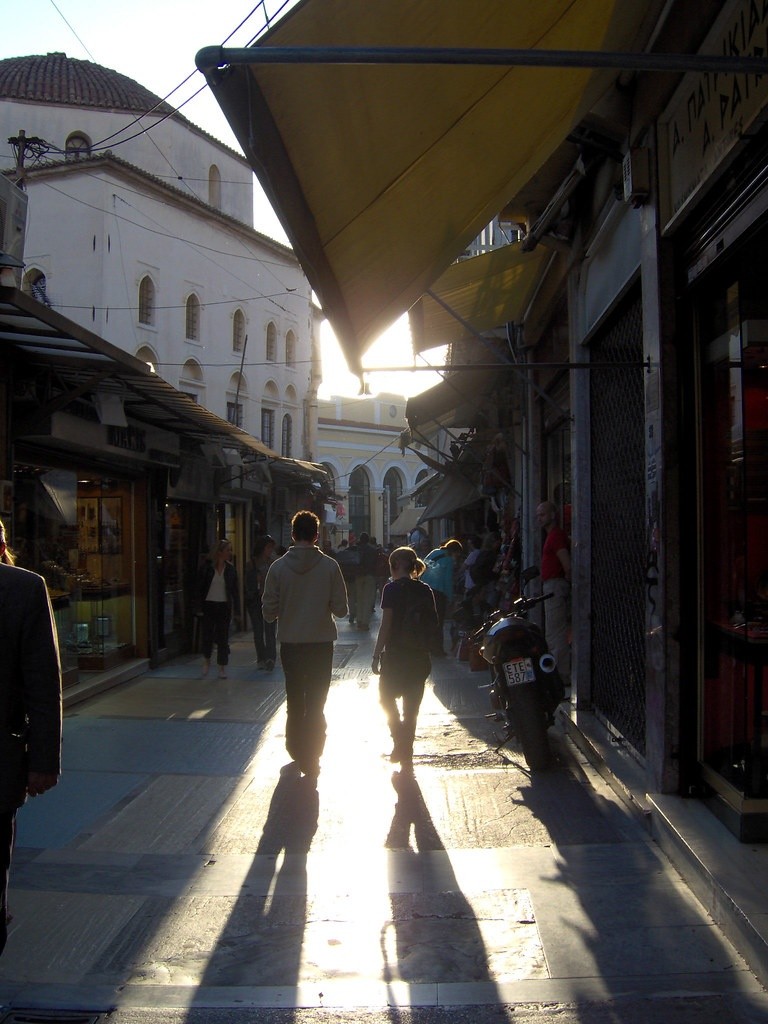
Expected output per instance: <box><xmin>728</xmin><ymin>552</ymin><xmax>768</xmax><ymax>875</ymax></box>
<box><xmin>466</xmin><ymin>567</ymin><xmax>567</xmax><ymax>772</ymax></box>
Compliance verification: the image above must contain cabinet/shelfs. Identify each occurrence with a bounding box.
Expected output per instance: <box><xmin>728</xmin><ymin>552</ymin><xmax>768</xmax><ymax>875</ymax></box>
<box><xmin>36</xmin><ymin>491</ymin><xmax>132</xmax><ymax>690</ymax></box>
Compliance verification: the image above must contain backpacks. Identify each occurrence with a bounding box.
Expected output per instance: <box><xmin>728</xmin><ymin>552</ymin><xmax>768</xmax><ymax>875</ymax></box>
<box><xmin>469</xmin><ymin>551</ymin><xmax>495</xmax><ymax>576</ymax></box>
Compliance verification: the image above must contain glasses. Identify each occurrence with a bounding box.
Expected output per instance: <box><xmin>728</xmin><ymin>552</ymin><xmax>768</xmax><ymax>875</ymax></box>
<box><xmin>266</xmin><ymin>534</ymin><xmax>271</xmax><ymax>539</ymax></box>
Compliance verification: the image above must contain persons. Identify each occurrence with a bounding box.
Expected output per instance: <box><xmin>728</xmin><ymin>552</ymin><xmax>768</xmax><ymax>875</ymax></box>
<box><xmin>340</xmin><ymin>533</ymin><xmax>395</xmax><ymax>632</ymax></box>
<box><xmin>183</xmin><ymin>537</ymin><xmax>242</xmax><ymax>681</ymax></box>
<box><xmin>460</xmin><ymin>535</ymin><xmax>482</xmax><ymax>599</ymax></box>
<box><xmin>0</xmin><ymin>518</ymin><xmax>66</xmax><ymax>962</ymax></box>
<box><xmin>420</xmin><ymin>537</ymin><xmax>463</xmax><ymax>659</ymax></box>
<box><xmin>412</xmin><ymin>538</ymin><xmax>432</xmax><ymax>559</ymax></box>
<box><xmin>259</xmin><ymin>509</ymin><xmax>349</xmax><ymax>777</ymax></box>
<box><xmin>244</xmin><ymin>535</ymin><xmax>278</xmax><ymax>671</ymax></box>
<box><xmin>322</xmin><ymin>540</ymin><xmax>335</xmax><ymax>557</ymax></box>
<box><xmin>336</xmin><ymin>538</ymin><xmax>348</xmax><ymax>553</ymax></box>
<box><xmin>534</xmin><ymin>501</ymin><xmax>572</xmax><ymax>703</ymax></box>
<box><xmin>370</xmin><ymin>546</ymin><xmax>446</xmax><ymax>766</ymax></box>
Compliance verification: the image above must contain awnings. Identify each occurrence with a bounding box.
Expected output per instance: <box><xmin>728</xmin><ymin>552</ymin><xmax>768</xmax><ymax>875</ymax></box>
<box><xmin>279</xmin><ymin>456</ymin><xmax>329</xmax><ymax>480</ymax></box>
<box><xmin>384</xmin><ymin>372</ymin><xmax>524</xmax><ymax>536</ymax></box>
<box><xmin>193</xmin><ymin>0</ymin><xmax>667</xmax><ymax>378</ymax></box>
<box><xmin>0</xmin><ymin>282</ymin><xmax>280</xmax><ymax>477</ymax></box>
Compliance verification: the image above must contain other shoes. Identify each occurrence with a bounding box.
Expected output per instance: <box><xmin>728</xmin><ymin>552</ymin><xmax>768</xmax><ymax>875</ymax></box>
<box><xmin>349</xmin><ymin>613</ymin><xmax>354</xmax><ymax>623</ymax></box>
<box><xmin>200</xmin><ymin>657</ymin><xmax>210</xmax><ymax>674</ymax></box>
<box><xmin>357</xmin><ymin>622</ymin><xmax>362</xmax><ymax>628</ymax></box>
<box><xmin>257</xmin><ymin>660</ymin><xmax>265</xmax><ymax>668</ymax></box>
<box><xmin>361</xmin><ymin>625</ymin><xmax>369</xmax><ymax>630</ymax></box>
<box><xmin>217</xmin><ymin>665</ymin><xmax>227</xmax><ymax>679</ymax></box>
